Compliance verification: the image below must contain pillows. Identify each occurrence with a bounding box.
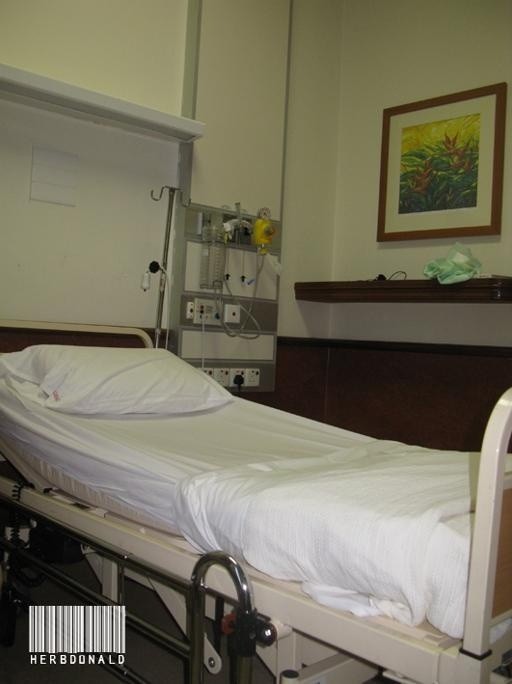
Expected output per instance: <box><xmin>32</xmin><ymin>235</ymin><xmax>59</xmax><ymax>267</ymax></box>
<box><xmin>1</xmin><ymin>343</ymin><xmax>236</xmax><ymax>416</ymax></box>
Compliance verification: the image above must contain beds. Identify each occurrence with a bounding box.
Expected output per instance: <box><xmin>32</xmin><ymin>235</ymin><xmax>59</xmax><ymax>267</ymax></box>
<box><xmin>0</xmin><ymin>319</ymin><xmax>512</xmax><ymax>683</ymax></box>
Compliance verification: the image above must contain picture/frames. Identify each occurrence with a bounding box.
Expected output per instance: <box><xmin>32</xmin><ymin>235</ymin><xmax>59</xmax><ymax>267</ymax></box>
<box><xmin>377</xmin><ymin>82</ymin><xmax>506</xmax><ymax>242</ymax></box>
